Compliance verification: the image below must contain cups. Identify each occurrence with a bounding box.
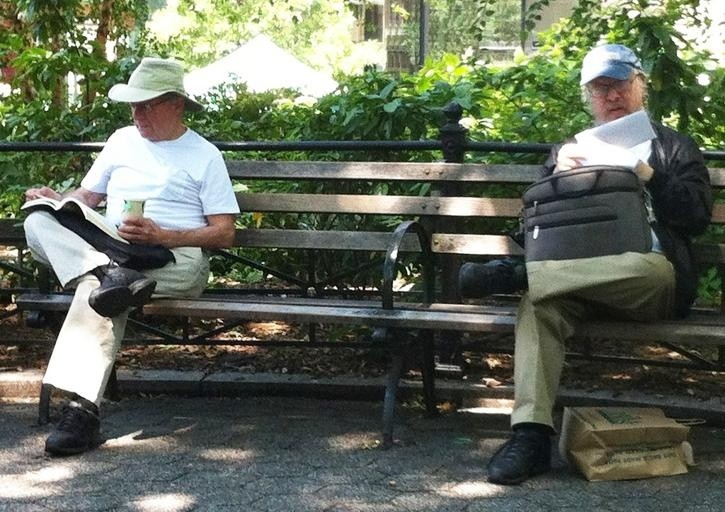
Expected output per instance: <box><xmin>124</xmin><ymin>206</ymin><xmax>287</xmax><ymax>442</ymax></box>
<box><xmin>124</xmin><ymin>198</ymin><xmax>146</xmax><ymax>220</ymax></box>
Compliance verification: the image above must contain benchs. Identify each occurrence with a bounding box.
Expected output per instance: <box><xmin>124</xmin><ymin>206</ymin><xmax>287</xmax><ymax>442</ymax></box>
<box><xmin>0</xmin><ymin>154</ymin><xmax>725</xmax><ymax>453</ymax></box>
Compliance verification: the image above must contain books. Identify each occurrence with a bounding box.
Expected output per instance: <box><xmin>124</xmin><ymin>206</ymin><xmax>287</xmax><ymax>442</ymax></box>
<box><xmin>20</xmin><ymin>196</ymin><xmax>132</xmax><ymax>245</ymax></box>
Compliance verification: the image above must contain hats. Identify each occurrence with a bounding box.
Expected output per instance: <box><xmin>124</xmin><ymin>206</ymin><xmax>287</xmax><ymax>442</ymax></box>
<box><xmin>580</xmin><ymin>44</ymin><xmax>642</xmax><ymax>86</ymax></box>
<box><xmin>108</xmin><ymin>57</ymin><xmax>204</xmax><ymax>114</ymax></box>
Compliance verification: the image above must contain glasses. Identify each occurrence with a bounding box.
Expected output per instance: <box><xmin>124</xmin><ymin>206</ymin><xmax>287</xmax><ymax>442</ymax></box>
<box><xmin>128</xmin><ymin>94</ymin><xmax>175</xmax><ymax>112</ymax></box>
<box><xmin>589</xmin><ymin>74</ymin><xmax>639</xmax><ymax>98</ymax></box>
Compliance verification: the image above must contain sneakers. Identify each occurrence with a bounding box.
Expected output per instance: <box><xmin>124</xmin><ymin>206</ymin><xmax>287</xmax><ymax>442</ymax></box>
<box><xmin>458</xmin><ymin>259</ymin><xmax>525</xmax><ymax>299</ymax></box>
<box><xmin>89</xmin><ymin>266</ymin><xmax>158</xmax><ymax>318</ymax></box>
<box><xmin>486</xmin><ymin>428</ymin><xmax>552</xmax><ymax>485</ymax></box>
<box><xmin>44</xmin><ymin>400</ymin><xmax>100</xmax><ymax>454</ymax></box>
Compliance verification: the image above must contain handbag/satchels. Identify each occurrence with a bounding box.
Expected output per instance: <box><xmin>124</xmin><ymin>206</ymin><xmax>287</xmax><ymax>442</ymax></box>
<box><xmin>49</xmin><ymin>207</ymin><xmax>170</xmax><ymax>272</ymax></box>
<box><xmin>559</xmin><ymin>406</ymin><xmax>708</xmax><ymax>482</ymax></box>
<box><xmin>521</xmin><ymin>165</ymin><xmax>654</xmax><ymax>263</ymax></box>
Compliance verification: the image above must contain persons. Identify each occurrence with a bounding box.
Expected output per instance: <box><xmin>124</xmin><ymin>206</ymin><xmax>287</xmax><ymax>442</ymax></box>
<box><xmin>458</xmin><ymin>44</ymin><xmax>714</xmax><ymax>484</ymax></box>
<box><xmin>23</xmin><ymin>57</ymin><xmax>242</xmax><ymax>454</ymax></box>
